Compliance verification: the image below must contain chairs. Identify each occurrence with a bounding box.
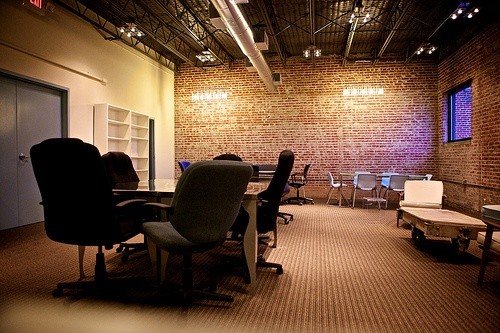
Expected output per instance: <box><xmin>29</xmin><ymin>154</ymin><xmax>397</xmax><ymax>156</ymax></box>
<box><xmin>326</xmin><ymin>170</ymin><xmax>432</xmax><ymax>210</ymax></box>
<box><xmin>397</xmin><ymin>180</ymin><xmax>448</xmax><ymax>227</ymax></box>
<box><xmin>477</xmin><ymin>205</ymin><xmax>500</xmax><ymax>288</ymax></box>
<box><xmin>30</xmin><ymin>138</ymin><xmax>315</xmax><ymax>313</ymax></box>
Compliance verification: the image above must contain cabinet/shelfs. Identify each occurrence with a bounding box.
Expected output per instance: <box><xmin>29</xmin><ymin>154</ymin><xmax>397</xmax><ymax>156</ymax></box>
<box><xmin>94</xmin><ymin>103</ymin><xmax>149</xmax><ymax>189</ymax></box>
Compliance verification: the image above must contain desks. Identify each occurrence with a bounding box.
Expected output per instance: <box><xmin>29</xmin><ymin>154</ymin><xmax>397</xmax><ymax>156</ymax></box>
<box><xmin>339</xmin><ymin>172</ymin><xmax>428</xmax><ymax>207</ymax></box>
<box><xmin>112</xmin><ymin>179</ymin><xmax>270</xmax><ymax>296</ymax></box>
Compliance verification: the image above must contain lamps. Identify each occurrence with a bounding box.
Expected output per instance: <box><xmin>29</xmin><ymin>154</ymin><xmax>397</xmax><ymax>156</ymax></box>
<box><xmin>417</xmin><ymin>42</ymin><xmax>438</xmax><ymax>55</ymax></box>
<box><xmin>196</xmin><ymin>46</ymin><xmax>216</xmax><ymax>63</ymax></box>
<box><xmin>117</xmin><ymin>17</ymin><xmax>146</xmax><ymax>38</ymax></box>
<box><xmin>348</xmin><ymin>0</ymin><xmax>370</xmax><ymax>23</ymax></box>
<box><xmin>302</xmin><ymin>43</ymin><xmax>321</xmax><ymax>58</ymax></box>
<box><xmin>450</xmin><ymin>6</ymin><xmax>480</xmax><ymax>20</ymax></box>
